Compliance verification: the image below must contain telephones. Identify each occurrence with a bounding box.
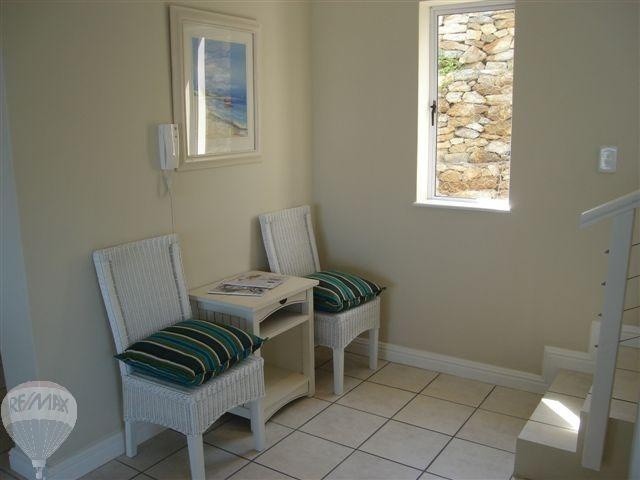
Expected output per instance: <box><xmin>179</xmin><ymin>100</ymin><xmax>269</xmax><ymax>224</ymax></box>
<box><xmin>158</xmin><ymin>124</ymin><xmax>179</xmax><ymax>170</ymax></box>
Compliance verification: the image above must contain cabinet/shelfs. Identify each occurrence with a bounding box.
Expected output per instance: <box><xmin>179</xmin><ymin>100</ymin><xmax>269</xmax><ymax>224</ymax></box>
<box><xmin>189</xmin><ymin>270</ymin><xmax>319</xmax><ymax>432</ymax></box>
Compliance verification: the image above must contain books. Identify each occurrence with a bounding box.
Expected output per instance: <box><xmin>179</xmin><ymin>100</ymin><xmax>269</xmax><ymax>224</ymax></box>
<box><xmin>206</xmin><ymin>274</ymin><xmax>290</xmax><ymax>296</ymax></box>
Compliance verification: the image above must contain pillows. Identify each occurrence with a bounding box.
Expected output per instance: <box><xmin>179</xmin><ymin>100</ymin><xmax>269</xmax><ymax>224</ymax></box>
<box><xmin>113</xmin><ymin>318</ymin><xmax>268</xmax><ymax>389</ymax></box>
<box><xmin>302</xmin><ymin>270</ymin><xmax>387</xmax><ymax>312</ymax></box>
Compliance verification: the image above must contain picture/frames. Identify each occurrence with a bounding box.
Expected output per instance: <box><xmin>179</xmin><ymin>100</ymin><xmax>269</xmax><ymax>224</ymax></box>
<box><xmin>170</xmin><ymin>5</ymin><xmax>263</xmax><ymax>172</ymax></box>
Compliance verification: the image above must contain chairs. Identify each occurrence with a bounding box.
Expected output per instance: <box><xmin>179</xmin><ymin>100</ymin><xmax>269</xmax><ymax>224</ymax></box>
<box><xmin>92</xmin><ymin>233</ymin><xmax>265</xmax><ymax>480</ymax></box>
<box><xmin>259</xmin><ymin>205</ymin><xmax>381</xmax><ymax>395</ymax></box>
